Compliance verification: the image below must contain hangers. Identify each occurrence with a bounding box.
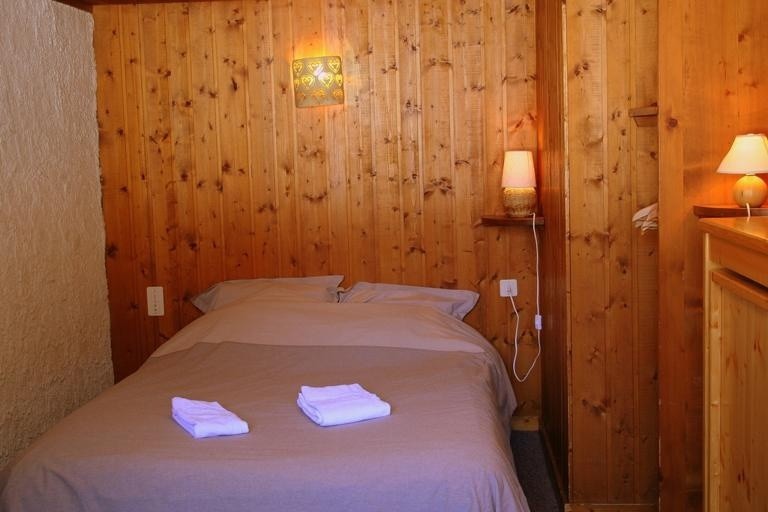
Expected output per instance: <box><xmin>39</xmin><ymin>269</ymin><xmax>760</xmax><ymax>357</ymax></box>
<box><xmin>631</xmin><ymin>202</ymin><xmax>658</xmax><ymax>235</ymax></box>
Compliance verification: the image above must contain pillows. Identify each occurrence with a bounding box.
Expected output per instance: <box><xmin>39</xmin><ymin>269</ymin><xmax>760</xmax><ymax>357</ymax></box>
<box><xmin>193</xmin><ymin>275</ymin><xmax>342</xmax><ymax>314</ymax></box>
<box><xmin>343</xmin><ymin>282</ymin><xmax>479</xmax><ymax>321</ymax></box>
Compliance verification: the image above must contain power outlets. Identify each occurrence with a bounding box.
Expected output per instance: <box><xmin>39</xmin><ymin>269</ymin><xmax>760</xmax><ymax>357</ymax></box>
<box><xmin>499</xmin><ymin>279</ymin><xmax>518</xmax><ymax>297</ymax></box>
<box><xmin>146</xmin><ymin>285</ymin><xmax>165</xmax><ymax>316</ymax></box>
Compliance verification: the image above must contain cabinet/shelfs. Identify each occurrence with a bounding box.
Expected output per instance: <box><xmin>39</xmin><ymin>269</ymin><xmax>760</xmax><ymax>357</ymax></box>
<box><xmin>534</xmin><ymin>0</ymin><xmax>569</xmax><ymax>508</ymax></box>
<box><xmin>697</xmin><ymin>218</ymin><xmax>768</xmax><ymax>510</ymax></box>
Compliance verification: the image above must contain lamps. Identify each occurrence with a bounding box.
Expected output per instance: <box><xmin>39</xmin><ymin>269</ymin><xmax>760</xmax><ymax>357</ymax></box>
<box><xmin>501</xmin><ymin>150</ymin><xmax>536</xmax><ymax>216</ymax></box>
<box><xmin>718</xmin><ymin>131</ymin><xmax>768</xmax><ymax>202</ymax></box>
<box><xmin>292</xmin><ymin>55</ymin><xmax>344</xmax><ymax>109</ymax></box>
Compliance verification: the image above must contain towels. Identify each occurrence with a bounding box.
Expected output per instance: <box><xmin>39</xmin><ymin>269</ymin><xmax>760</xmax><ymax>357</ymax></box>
<box><xmin>295</xmin><ymin>383</ymin><xmax>391</xmax><ymax>426</ymax></box>
<box><xmin>172</xmin><ymin>397</ymin><xmax>250</xmax><ymax>439</ymax></box>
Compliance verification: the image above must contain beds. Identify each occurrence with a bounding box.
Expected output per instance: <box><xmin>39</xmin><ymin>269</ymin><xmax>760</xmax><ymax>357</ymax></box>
<box><xmin>3</xmin><ymin>272</ymin><xmax>531</xmax><ymax>511</ymax></box>
